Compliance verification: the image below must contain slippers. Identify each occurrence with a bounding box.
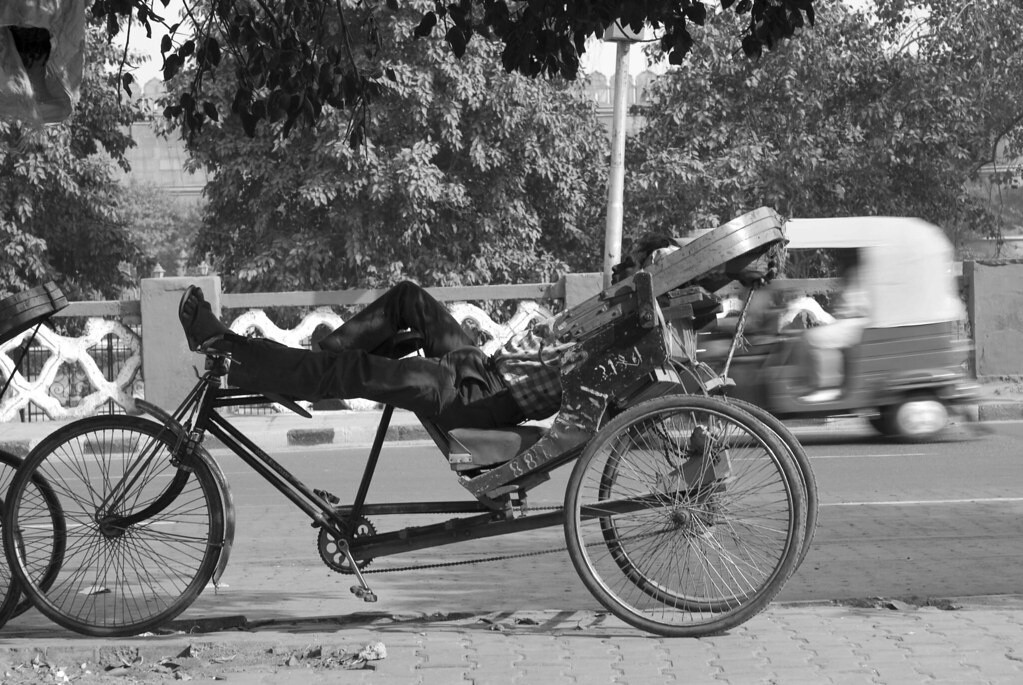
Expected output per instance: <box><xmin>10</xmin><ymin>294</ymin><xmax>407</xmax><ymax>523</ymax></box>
<box><xmin>179</xmin><ymin>285</ymin><xmax>212</xmax><ymax>352</ymax></box>
<box><xmin>264</xmin><ymin>392</ymin><xmax>312</xmax><ymax>418</ymax></box>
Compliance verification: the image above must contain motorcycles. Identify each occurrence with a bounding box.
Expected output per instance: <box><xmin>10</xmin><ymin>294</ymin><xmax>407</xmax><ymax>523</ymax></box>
<box><xmin>649</xmin><ymin>215</ymin><xmax>980</xmax><ymax>442</ymax></box>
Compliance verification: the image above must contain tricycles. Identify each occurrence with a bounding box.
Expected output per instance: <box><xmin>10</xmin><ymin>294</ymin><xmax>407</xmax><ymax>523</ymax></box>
<box><xmin>0</xmin><ymin>207</ymin><xmax>821</xmax><ymax>638</ymax></box>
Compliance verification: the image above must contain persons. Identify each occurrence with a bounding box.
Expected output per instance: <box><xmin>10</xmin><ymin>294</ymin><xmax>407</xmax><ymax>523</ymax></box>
<box><xmin>796</xmin><ymin>259</ymin><xmax>868</xmax><ymax>403</ymax></box>
<box><xmin>178</xmin><ymin>232</ymin><xmax>680</xmax><ymax>429</ymax></box>
